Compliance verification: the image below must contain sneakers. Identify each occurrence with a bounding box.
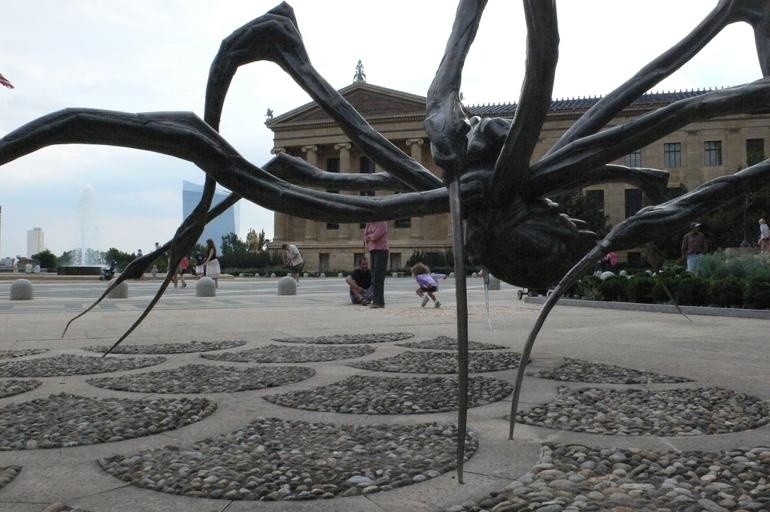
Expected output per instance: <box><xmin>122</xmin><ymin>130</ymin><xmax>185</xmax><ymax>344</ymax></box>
<box><xmin>421</xmin><ymin>296</ymin><xmax>441</xmax><ymax>309</ymax></box>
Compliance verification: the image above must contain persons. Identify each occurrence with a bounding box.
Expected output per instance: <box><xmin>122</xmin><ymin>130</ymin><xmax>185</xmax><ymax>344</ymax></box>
<box><xmin>681</xmin><ymin>222</ymin><xmax>708</xmax><ymax>273</ymax></box>
<box><xmin>346</xmin><ymin>257</ymin><xmax>374</xmax><ymax>306</ymax></box>
<box><xmin>363</xmin><ymin>221</ymin><xmax>390</xmax><ymax>308</ymax></box>
<box><xmin>33</xmin><ymin>261</ymin><xmax>40</xmax><ymax>272</ymax></box>
<box><xmin>608</xmin><ymin>251</ymin><xmax>617</xmax><ymax>270</ymax></box>
<box><xmin>137</xmin><ymin>249</ymin><xmax>145</xmax><ymax>280</ymax></box>
<box><xmin>282</xmin><ymin>244</ymin><xmax>304</xmax><ymax>286</ymax></box>
<box><xmin>201</xmin><ymin>239</ymin><xmax>220</xmax><ymax>288</ymax></box>
<box><xmin>13</xmin><ymin>256</ymin><xmax>21</xmax><ymax>273</ymax></box>
<box><xmin>152</xmin><ymin>265</ymin><xmax>158</xmax><ymax>279</ymax></box>
<box><xmin>410</xmin><ymin>262</ymin><xmax>448</xmax><ymax>308</ymax></box>
<box><xmin>195</xmin><ymin>250</ymin><xmax>206</xmax><ymax>279</ymax></box>
<box><xmin>757</xmin><ymin>218</ymin><xmax>770</xmax><ymax>253</ymax></box>
<box><xmin>25</xmin><ymin>261</ymin><xmax>32</xmax><ymax>273</ymax></box>
<box><xmin>180</xmin><ymin>257</ymin><xmax>189</xmax><ymax>288</ymax></box>
